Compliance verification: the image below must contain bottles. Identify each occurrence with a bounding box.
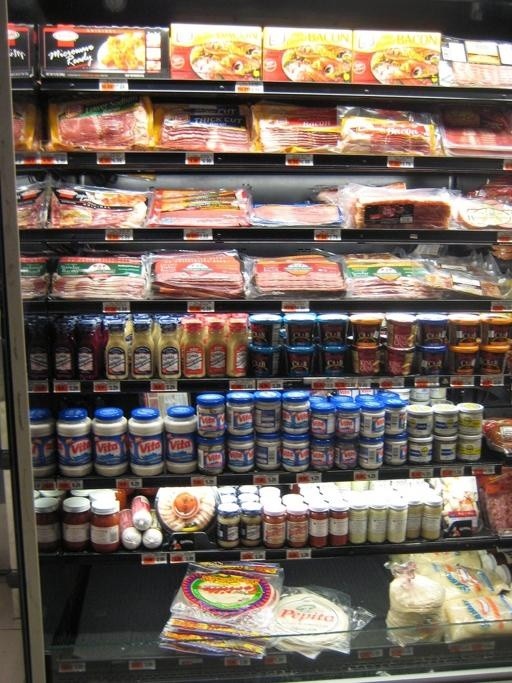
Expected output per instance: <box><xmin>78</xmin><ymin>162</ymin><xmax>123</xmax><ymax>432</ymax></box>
<box><xmin>24</xmin><ymin>314</ymin><xmax>249</xmax><ymax>385</ymax></box>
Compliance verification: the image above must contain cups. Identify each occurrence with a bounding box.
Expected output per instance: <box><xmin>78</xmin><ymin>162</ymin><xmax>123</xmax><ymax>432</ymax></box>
<box><xmin>247</xmin><ymin>310</ymin><xmax>512</xmax><ymax>379</ymax></box>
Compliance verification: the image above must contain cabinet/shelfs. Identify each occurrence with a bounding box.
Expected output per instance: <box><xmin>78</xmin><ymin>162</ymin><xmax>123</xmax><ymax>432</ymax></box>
<box><xmin>11</xmin><ymin>74</ymin><xmax>512</xmax><ymax>565</ymax></box>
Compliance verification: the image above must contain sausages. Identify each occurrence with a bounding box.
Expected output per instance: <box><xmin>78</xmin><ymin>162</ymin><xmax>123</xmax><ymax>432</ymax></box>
<box><xmin>120</xmin><ymin>495</ymin><xmax>164</xmax><ymax>550</ymax></box>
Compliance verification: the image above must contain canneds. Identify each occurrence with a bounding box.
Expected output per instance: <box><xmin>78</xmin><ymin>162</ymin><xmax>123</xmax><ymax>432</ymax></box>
<box><xmin>217</xmin><ymin>479</ymin><xmax>445</xmax><ymax>549</ymax></box>
<box><xmin>29</xmin><ymin>408</ymin><xmax>57</xmax><ymax>477</ymax></box>
<box><xmin>197</xmin><ymin>390</ymin><xmax>484</xmax><ymax>476</ymax></box>
<box><xmin>56</xmin><ymin>408</ymin><xmax>92</xmax><ymax>478</ymax></box>
<box><xmin>128</xmin><ymin>407</ymin><xmax>165</xmax><ymax>476</ymax></box>
<box><xmin>164</xmin><ymin>406</ymin><xmax>198</xmax><ymax>474</ymax></box>
<box><xmin>34</xmin><ymin>489</ymin><xmax>121</xmax><ymax>555</ymax></box>
<box><xmin>91</xmin><ymin>408</ymin><xmax>129</xmax><ymax>477</ymax></box>
